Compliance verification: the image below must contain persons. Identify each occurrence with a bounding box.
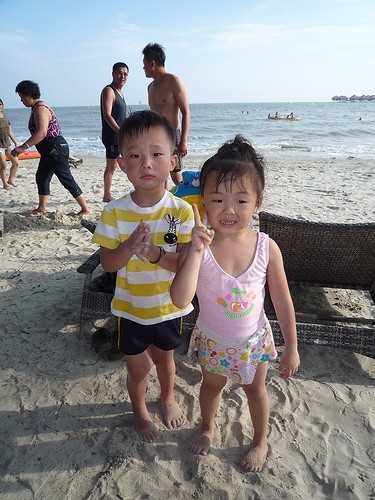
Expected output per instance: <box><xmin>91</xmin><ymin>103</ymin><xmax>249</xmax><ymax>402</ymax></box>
<box><xmin>100</xmin><ymin>62</ymin><xmax>129</xmax><ymax>202</ymax></box>
<box><xmin>170</xmin><ymin>136</ymin><xmax>300</xmax><ymax>472</ymax></box>
<box><xmin>11</xmin><ymin>80</ymin><xmax>92</xmax><ymax>218</ymax></box>
<box><xmin>0</xmin><ymin>99</ymin><xmax>20</xmax><ymax>190</ymax></box>
<box><xmin>142</xmin><ymin>42</ymin><xmax>189</xmax><ymax>191</ymax></box>
<box><xmin>92</xmin><ymin>111</ymin><xmax>195</xmax><ymax>443</ymax></box>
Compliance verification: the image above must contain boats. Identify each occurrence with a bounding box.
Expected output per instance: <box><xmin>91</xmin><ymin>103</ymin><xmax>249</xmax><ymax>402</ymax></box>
<box><xmin>4</xmin><ymin>150</ymin><xmax>41</xmax><ymax>161</ymax></box>
<box><xmin>268</xmin><ymin>115</ymin><xmax>303</xmax><ymax>121</ymax></box>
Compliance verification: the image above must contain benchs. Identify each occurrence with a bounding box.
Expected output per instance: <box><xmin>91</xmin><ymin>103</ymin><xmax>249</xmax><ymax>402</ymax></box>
<box><xmin>77</xmin><ymin>212</ymin><xmax>375</xmax><ymax>363</ymax></box>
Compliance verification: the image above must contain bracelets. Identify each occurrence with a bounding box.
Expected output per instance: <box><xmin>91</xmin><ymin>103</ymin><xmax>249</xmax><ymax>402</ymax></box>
<box><xmin>149</xmin><ymin>246</ymin><xmax>164</xmax><ymax>264</ymax></box>
<box><xmin>24</xmin><ymin>142</ymin><xmax>30</xmax><ymax>148</ymax></box>
